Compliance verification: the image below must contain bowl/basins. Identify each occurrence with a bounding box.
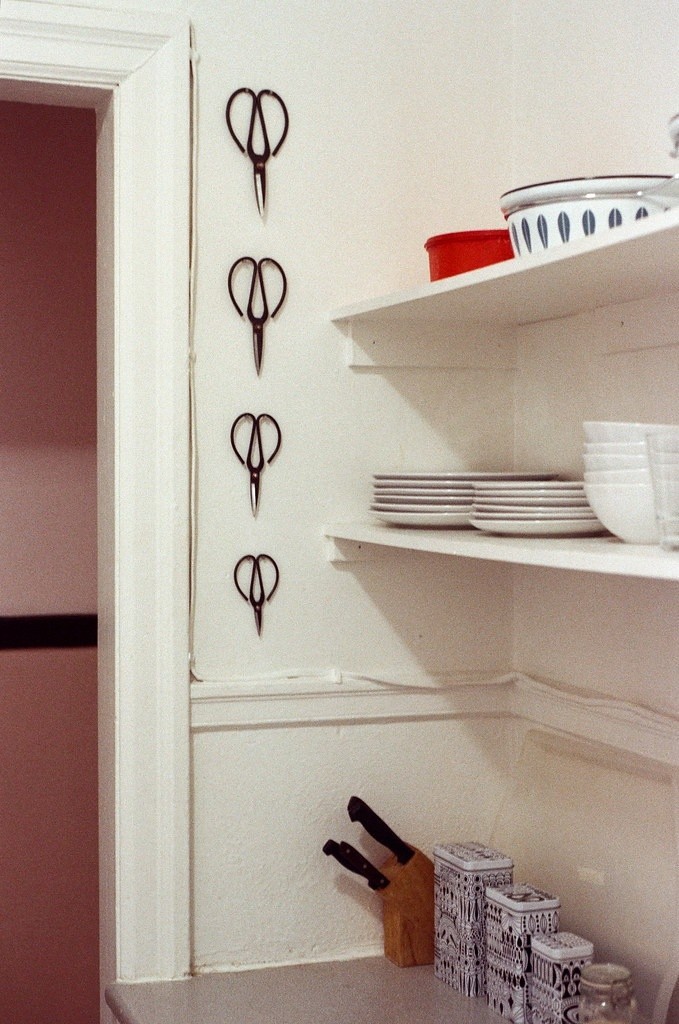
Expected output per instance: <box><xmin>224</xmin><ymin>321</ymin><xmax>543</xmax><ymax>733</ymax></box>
<box><xmin>500</xmin><ymin>174</ymin><xmax>672</xmax><ymax>255</ymax></box>
<box><xmin>423</xmin><ymin>228</ymin><xmax>515</xmax><ymax>282</ymax></box>
<box><xmin>582</xmin><ymin>421</ymin><xmax>679</xmax><ymax>543</ymax></box>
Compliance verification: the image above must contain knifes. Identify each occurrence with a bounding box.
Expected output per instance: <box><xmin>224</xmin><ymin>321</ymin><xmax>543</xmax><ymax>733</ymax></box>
<box><xmin>323</xmin><ymin>797</ymin><xmax>416</xmax><ymax>890</ymax></box>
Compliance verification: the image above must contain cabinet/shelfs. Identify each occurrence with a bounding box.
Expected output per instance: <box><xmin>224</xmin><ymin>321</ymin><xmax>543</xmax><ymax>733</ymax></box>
<box><xmin>325</xmin><ymin>204</ymin><xmax>679</xmax><ymax>581</ymax></box>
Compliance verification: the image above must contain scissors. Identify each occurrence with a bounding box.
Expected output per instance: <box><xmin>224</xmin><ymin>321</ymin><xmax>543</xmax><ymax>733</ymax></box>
<box><xmin>228</xmin><ymin>257</ymin><xmax>287</xmax><ymax>377</ymax></box>
<box><xmin>226</xmin><ymin>87</ymin><xmax>289</xmax><ymax>218</ymax></box>
<box><xmin>233</xmin><ymin>554</ymin><xmax>279</xmax><ymax>638</ymax></box>
<box><xmin>230</xmin><ymin>413</ymin><xmax>282</xmax><ymax>518</ymax></box>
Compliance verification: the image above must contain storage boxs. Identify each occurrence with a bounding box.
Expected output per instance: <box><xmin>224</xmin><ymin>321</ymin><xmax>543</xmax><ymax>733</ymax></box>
<box><xmin>531</xmin><ymin>931</ymin><xmax>594</xmax><ymax>1024</ymax></box>
<box><xmin>433</xmin><ymin>841</ymin><xmax>515</xmax><ymax>998</ymax></box>
<box><xmin>485</xmin><ymin>884</ymin><xmax>560</xmax><ymax>1024</ymax></box>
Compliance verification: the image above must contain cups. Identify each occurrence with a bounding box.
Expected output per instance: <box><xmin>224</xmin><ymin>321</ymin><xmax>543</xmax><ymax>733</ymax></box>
<box><xmin>644</xmin><ymin>430</ymin><xmax>679</xmax><ymax>551</ymax></box>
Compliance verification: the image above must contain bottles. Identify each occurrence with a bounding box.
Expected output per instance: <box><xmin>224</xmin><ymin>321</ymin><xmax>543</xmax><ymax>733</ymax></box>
<box><xmin>580</xmin><ymin>963</ymin><xmax>638</xmax><ymax>1024</ymax></box>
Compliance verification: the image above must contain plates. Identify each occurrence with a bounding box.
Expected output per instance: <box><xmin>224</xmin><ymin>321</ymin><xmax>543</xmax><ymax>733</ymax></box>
<box><xmin>368</xmin><ymin>472</ymin><xmax>608</xmax><ymax>533</ymax></box>
